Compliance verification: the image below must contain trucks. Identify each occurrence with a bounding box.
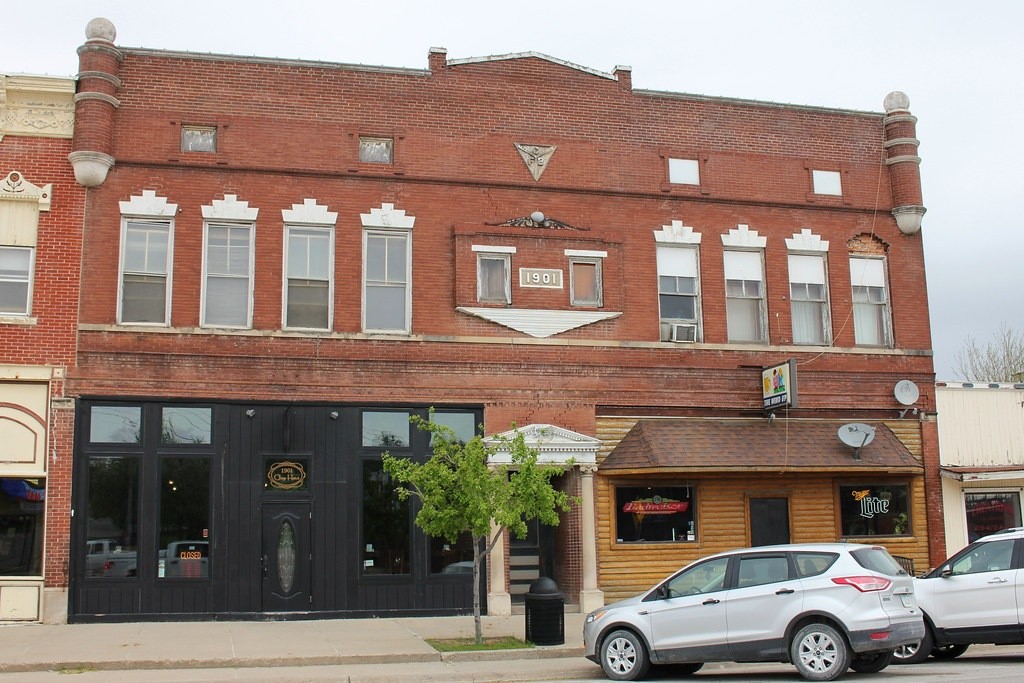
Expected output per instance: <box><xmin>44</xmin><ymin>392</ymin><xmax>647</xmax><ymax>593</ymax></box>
<box><xmin>84</xmin><ymin>541</ymin><xmax>137</xmax><ymax>577</ymax></box>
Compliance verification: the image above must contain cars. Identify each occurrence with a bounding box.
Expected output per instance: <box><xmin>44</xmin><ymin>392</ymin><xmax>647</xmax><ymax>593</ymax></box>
<box><xmin>162</xmin><ymin>542</ymin><xmax>210</xmax><ymax>579</ymax></box>
<box><xmin>583</xmin><ymin>541</ymin><xmax>923</xmax><ymax>682</ymax></box>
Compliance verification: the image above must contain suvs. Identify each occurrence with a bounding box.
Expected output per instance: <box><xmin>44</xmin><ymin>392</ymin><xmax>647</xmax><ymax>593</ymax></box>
<box><xmin>894</xmin><ymin>528</ymin><xmax>1024</xmax><ymax>664</ymax></box>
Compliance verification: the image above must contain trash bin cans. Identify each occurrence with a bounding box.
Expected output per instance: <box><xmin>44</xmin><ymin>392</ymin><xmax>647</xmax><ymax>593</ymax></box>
<box><xmin>523</xmin><ymin>576</ymin><xmax>567</xmax><ymax>645</ymax></box>
